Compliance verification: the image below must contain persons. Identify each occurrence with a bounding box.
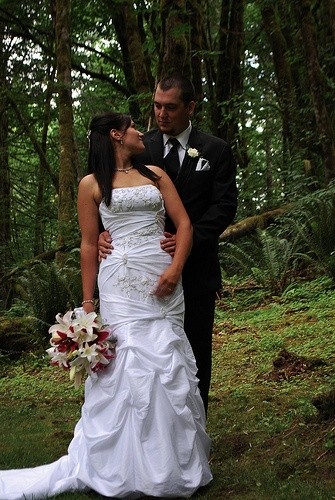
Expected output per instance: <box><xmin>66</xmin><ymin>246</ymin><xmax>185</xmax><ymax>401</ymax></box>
<box><xmin>66</xmin><ymin>111</ymin><xmax>215</xmax><ymax>499</ymax></box>
<box><xmin>98</xmin><ymin>72</ymin><xmax>238</xmax><ymax>425</ymax></box>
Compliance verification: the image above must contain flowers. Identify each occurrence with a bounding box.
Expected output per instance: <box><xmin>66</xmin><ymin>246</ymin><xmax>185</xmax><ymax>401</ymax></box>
<box><xmin>184</xmin><ymin>145</ymin><xmax>200</xmax><ymax>157</ymax></box>
<box><xmin>46</xmin><ymin>299</ymin><xmax>118</xmax><ymax>390</ymax></box>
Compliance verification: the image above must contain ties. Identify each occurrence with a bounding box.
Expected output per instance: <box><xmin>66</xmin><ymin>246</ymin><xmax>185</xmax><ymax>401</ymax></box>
<box><xmin>163</xmin><ymin>138</ymin><xmax>180</xmax><ymax>182</ymax></box>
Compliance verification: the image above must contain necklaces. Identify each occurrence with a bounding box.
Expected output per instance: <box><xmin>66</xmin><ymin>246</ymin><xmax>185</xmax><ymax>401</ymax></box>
<box><xmin>114</xmin><ymin>165</ymin><xmax>133</xmax><ymax>174</ymax></box>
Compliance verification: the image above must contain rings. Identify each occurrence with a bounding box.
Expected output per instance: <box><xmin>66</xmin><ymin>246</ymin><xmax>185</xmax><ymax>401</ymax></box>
<box><xmin>168</xmin><ymin>288</ymin><xmax>173</xmax><ymax>292</ymax></box>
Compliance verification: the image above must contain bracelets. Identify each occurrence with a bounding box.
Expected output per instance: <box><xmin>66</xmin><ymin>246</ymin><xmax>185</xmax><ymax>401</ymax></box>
<box><xmin>81</xmin><ymin>300</ymin><xmax>94</xmax><ymax>305</ymax></box>
